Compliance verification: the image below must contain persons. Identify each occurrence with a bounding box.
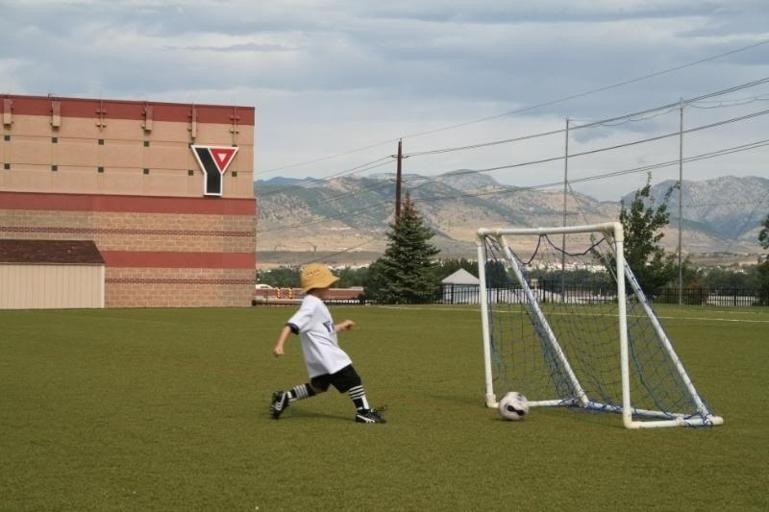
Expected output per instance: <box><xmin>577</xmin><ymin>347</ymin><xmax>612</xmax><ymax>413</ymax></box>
<box><xmin>270</xmin><ymin>262</ymin><xmax>386</xmax><ymax>424</ymax></box>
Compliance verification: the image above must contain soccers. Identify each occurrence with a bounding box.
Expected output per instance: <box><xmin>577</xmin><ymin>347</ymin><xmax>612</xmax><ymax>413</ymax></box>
<box><xmin>500</xmin><ymin>391</ymin><xmax>529</xmax><ymax>420</ymax></box>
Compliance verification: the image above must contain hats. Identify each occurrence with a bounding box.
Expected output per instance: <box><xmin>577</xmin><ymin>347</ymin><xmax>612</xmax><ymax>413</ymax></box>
<box><xmin>298</xmin><ymin>262</ymin><xmax>339</xmax><ymax>296</ymax></box>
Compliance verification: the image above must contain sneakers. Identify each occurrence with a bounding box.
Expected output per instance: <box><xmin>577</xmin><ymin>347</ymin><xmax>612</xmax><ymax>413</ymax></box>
<box><xmin>354</xmin><ymin>406</ymin><xmax>387</xmax><ymax>425</ymax></box>
<box><xmin>270</xmin><ymin>390</ymin><xmax>288</xmax><ymax>421</ymax></box>
